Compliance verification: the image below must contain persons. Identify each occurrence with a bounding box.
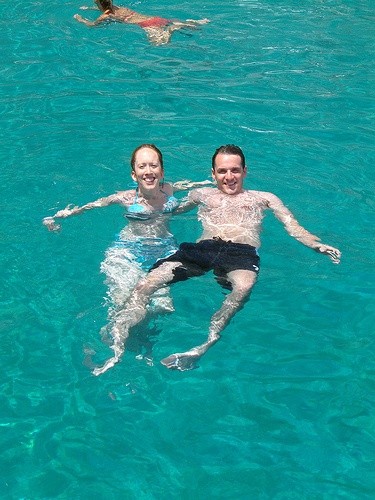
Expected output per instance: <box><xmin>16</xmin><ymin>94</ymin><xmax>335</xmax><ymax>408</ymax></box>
<box><xmin>42</xmin><ymin>143</ymin><xmax>220</xmax><ymax>342</ymax></box>
<box><xmin>89</xmin><ymin>143</ymin><xmax>342</xmax><ymax>377</ymax></box>
<box><xmin>72</xmin><ymin>0</ymin><xmax>211</xmax><ymax>47</ymax></box>
<box><xmin>90</xmin><ymin>143</ymin><xmax>342</xmax><ymax>377</ymax></box>
